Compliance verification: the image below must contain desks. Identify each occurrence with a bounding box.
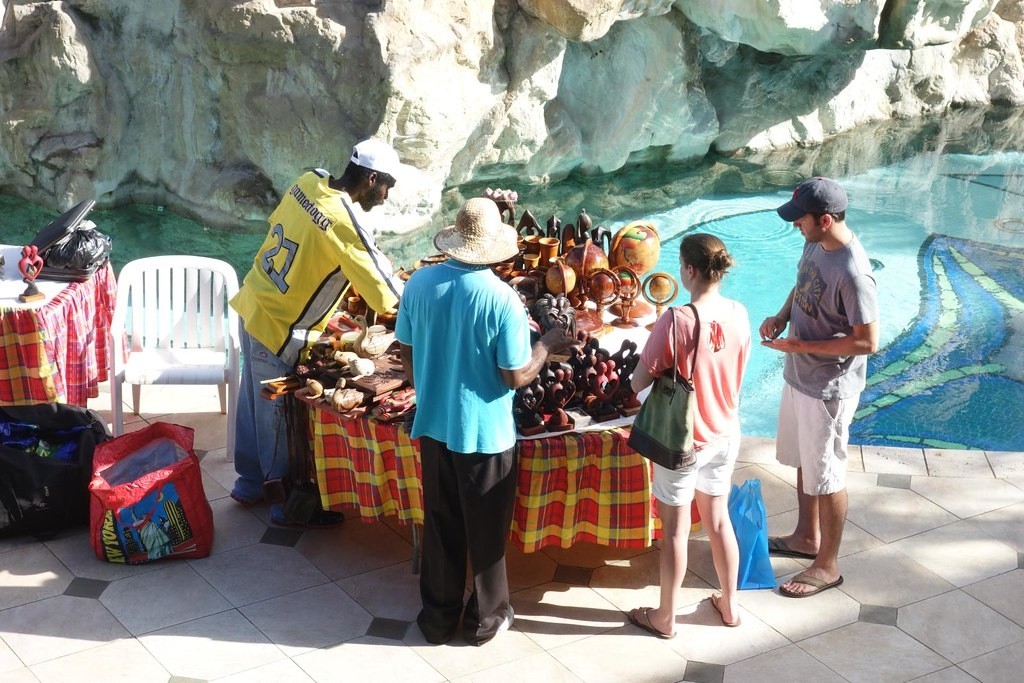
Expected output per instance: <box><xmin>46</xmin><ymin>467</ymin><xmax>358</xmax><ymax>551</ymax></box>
<box><xmin>301</xmin><ymin>291</ymin><xmax>703</xmax><ymax>578</ymax></box>
<box><xmin>1</xmin><ymin>243</ymin><xmax>131</xmax><ymax>409</ymax></box>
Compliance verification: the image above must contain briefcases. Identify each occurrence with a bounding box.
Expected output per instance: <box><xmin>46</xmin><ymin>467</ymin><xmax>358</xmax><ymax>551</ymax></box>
<box><xmin>27</xmin><ymin>200</ymin><xmax>109</xmax><ymax>282</ymax></box>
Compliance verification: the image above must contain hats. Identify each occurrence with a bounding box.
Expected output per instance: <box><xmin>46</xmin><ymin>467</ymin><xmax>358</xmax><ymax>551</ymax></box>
<box><xmin>351</xmin><ymin>138</ymin><xmax>418</xmax><ymax>179</ymax></box>
<box><xmin>434</xmin><ymin>197</ymin><xmax>520</xmax><ymax>266</ymax></box>
<box><xmin>777</xmin><ymin>177</ymin><xmax>848</xmax><ymax>222</ymax></box>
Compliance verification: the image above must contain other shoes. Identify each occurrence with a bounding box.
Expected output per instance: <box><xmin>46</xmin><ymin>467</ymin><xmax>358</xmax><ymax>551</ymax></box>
<box><xmin>230</xmin><ymin>487</ymin><xmax>268</xmax><ymax>506</ymax></box>
<box><xmin>273</xmin><ymin>508</ymin><xmax>345</xmax><ymax>529</ymax></box>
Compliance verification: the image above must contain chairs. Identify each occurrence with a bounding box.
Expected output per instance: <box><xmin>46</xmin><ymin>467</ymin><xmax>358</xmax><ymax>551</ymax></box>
<box><xmin>106</xmin><ymin>254</ymin><xmax>244</xmax><ymax>464</ymax></box>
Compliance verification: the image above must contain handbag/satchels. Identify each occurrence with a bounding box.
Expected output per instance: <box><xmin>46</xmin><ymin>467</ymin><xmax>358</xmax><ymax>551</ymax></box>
<box><xmin>0</xmin><ymin>401</ymin><xmax>116</xmax><ymax>540</ymax></box>
<box><xmin>627</xmin><ymin>369</ymin><xmax>699</xmax><ymax>471</ymax></box>
<box><xmin>86</xmin><ymin>421</ymin><xmax>215</xmax><ymax>565</ymax></box>
<box><xmin>728</xmin><ymin>478</ymin><xmax>776</xmax><ymax>590</ymax></box>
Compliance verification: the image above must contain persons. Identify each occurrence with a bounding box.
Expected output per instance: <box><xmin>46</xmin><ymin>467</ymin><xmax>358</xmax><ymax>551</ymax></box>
<box><xmin>228</xmin><ymin>139</ymin><xmax>410</xmax><ymax>530</ymax></box>
<box><xmin>628</xmin><ymin>233</ymin><xmax>753</xmax><ymax>641</ymax></box>
<box><xmin>757</xmin><ymin>174</ymin><xmax>879</xmax><ymax>599</ymax></box>
<box><xmin>392</xmin><ymin>197</ymin><xmax>583</xmax><ymax>652</ymax></box>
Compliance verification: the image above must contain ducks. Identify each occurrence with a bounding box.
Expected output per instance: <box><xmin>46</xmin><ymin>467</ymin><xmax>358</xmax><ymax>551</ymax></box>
<box><xmin>350</xmin><ymin>315</ymin><xmax>398</xmax><ymax>359</ymax></box>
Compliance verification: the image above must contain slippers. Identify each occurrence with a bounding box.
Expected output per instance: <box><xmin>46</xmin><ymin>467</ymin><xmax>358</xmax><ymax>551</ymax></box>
<box><xmin>778</xmin><ymin>573</ymin><xmax>844</xmax><ymax>598</ymax></box>
<box><xmin>629</xmin><ymin>607</ymin><xmax>677</xmax><ymax>639</ymax></box>
<box><xmin>712</xmin><ymin>595</ymin><xmax>742</xmax><ymax>627</ymax></box>
<box><xmin>768</xmin><ymin>535</ymin><xmax>819</xmax><ymax>560</ymax></box>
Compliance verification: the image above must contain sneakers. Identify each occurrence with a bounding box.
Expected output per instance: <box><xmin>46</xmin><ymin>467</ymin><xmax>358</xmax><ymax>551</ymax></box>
<box><xmin>480</xmin><ymin>606</ymin><xmax>515</xmax><ymax>644</ymax></box>
